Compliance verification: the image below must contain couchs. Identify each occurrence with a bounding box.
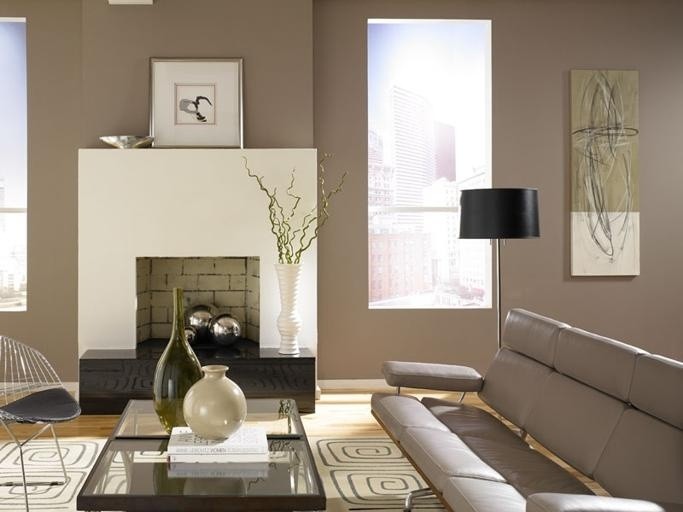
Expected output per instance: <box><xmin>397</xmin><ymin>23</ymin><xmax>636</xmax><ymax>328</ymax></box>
<box><xmin>368</xmin><ymin>305</ymin><xmax>683</xmax><ymax>512</ymax></box>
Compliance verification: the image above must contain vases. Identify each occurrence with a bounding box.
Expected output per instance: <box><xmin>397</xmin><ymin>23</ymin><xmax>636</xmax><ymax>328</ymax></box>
<box><xmin>150</xmin><ymin>283</ymin><xmax>205</xmax><ymax>437</ymax></box>
<box><xmin>180</xmin><ymin>362</ymin><xmax>248</xmax><ymax>443</ymax></box>
<box><xmin>178</xmin><ymin>474</ymin><xmax>248</xmax><ymax>499</ymax></box>
<box><xmin>277</xmin><ymin>263</ymin><xmax>307</xmax><ymax>354</ymax></box>
<box><xmin>152</xmin><ymin>437</ymin><xmax>185</xmax><ymax>497</ymax></box>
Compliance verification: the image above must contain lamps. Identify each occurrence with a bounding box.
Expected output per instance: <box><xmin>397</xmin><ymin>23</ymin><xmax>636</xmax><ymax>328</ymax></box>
<box><xmin>455</xmin><ymin>185</ymin><xmax>542</xmax><ymax>355</ymax></box>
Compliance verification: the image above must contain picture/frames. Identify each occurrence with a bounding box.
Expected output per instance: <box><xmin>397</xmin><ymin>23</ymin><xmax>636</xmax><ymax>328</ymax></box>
<box><xmin>147</xmin><ymin>56</ymin><xmax>245</xmax><ymax>150</ymax></box>
<box><xmin>568</xmin><ymin>69</ymin><xmax>641</xmax><ymax>280</ymax></box>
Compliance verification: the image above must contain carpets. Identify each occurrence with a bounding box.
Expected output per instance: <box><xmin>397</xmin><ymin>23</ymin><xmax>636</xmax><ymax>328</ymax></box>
<box><xmin>0</xmin><ymin>434</ymin><xmax>446</xmax><ymax>511</ymax></box>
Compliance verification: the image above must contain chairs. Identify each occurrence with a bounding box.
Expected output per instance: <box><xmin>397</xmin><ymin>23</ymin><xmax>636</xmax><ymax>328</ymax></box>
<box><xmin>0</xmin><ymin>331</ymin><xmax>84</xmax><ymax>511</ymax></box>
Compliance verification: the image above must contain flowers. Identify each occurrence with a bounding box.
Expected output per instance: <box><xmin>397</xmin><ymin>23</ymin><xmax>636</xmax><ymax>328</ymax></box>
<box><xmin>243</xmin><ymin>149</ymin><xmax>347</xmax><ymax>263</ymax></box>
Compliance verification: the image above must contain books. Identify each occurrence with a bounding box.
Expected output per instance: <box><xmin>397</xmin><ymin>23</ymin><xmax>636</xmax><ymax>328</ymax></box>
<box><xmin>167</xmin><ymin>463</ymin><xmax>269</xmax><ymax>479</ymax></box>
<box><xmin>167</xmin><ymin>427</ymin><xmax>269</xmax><ymax>462</ymax></box>
